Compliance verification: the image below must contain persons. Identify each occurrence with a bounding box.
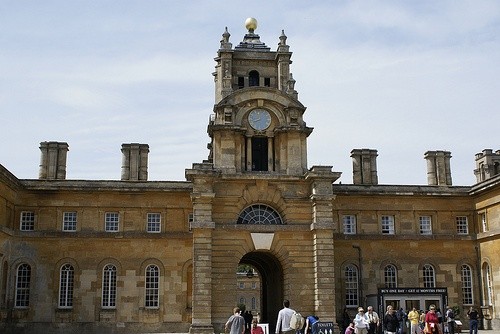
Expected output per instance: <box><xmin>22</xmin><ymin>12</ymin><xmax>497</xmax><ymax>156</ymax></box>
<box><xmin>304</xmin><ymin>315</ymin><xmax>319</xmax><ymax>334</ymax></box>
<box><xmin>365</xmin><ymin>306</ymin><xmax>380</xmax><ymax>334</ymax></box>
<box><xmin>466</xmin><ymin>306</ymin><xmax>479</xmax><ymax>334</ymax></box>
<box><xmin>353</xmin><ymin>307</ymin><xmax>368</xmax><ymax>334</ymax></box>
<box><xmin>345</xmin><ymin>323</ymin><xmax>354</xmax><ymax>334</ymax></box>
<box><xmin>244</xmin><ymin>311</ymin><xmax>253</xmax><ymax>329</ymax></box>
<box><xmin>408</xmin><ymin>304</ymin><xmax>443</xmax><ymax>334</ymax></box>
<box><xmin>225</xmin><ymin>307</ymin><xmax>245</xmax><ymax>334</ymax></box>
<box><xmin>250</xmin><ymin>318</ymin><xmax>264</xmax><ymax>334</ymax></box>
<box><xmin>383</xmin><ymin>305</ymin><xmax>400</xmax><ymax>334</ymax></box>
<box><xmin>445</xmin><ymin>306</ymin><xmax>454</xmax><ymax>334</ymax></box>
<box><xmin>396</xmin><ymin>308</ymin><xmax>407</xmax><ymax>334</ymax></box>
<box><xmin>275</xmin><ymin>299</ymin><xmax>296</xmax><ymax>334</ymax></box>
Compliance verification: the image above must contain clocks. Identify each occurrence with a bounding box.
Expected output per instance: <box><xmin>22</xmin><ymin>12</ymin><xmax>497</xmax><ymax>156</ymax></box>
<box><xmin>248</xmin><ymin>109</ymin><xmax>272</xmax><ymax>130</ymax></box>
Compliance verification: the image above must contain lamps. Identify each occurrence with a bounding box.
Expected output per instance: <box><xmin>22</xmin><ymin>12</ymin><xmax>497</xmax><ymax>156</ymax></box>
<box><xmin>246</xmin><ymin>268</ymin><xmax>254</xmax><ymax>278</ymax></box>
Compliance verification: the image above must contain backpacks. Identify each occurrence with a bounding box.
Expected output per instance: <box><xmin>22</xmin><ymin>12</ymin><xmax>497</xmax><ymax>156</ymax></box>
<box><xmin>288</xmin><ymin>310</ymin><xmax>303</xmax><ymax>329</ymax></box>
